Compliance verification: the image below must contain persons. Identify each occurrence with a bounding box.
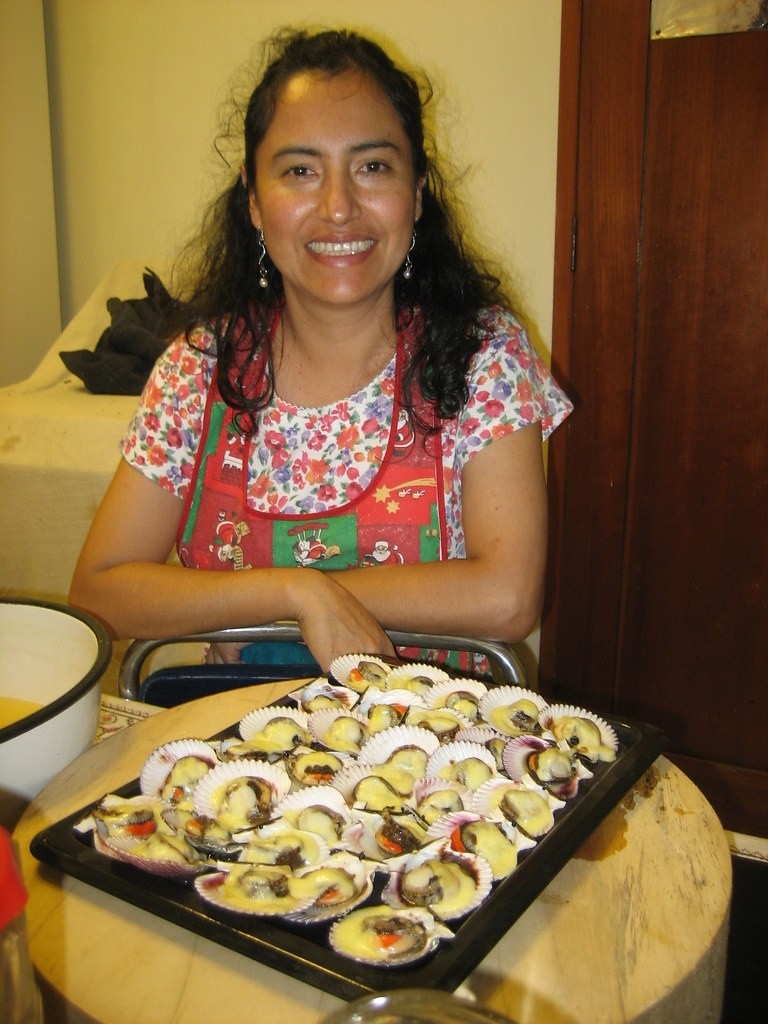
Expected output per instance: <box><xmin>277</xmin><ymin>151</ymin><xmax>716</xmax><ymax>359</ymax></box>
<box><xmin>65</xmin><ymin>24</ymin><xmax>550</xmax><ymax>678</ymax></box>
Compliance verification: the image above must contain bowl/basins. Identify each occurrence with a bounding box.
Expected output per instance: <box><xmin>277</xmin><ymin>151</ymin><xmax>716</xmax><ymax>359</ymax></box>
<box><xmin>0</xmin><ymin>598</ymin><xmax>112</xmax><ymax>821</ymax></box>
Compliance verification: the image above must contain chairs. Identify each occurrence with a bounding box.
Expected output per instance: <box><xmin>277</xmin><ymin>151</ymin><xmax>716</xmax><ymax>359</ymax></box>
<box><xmin>118</xmin><ymin>616</ymin><xmax>527</xmax><ymax>705</ymax></box>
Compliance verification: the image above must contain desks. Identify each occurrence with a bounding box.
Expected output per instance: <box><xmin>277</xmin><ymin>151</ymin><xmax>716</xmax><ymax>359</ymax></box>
<box><xmin>0</xmin><ymin>753</ymin><xmax>768</xmax><ymax>1023</ymax></box>
